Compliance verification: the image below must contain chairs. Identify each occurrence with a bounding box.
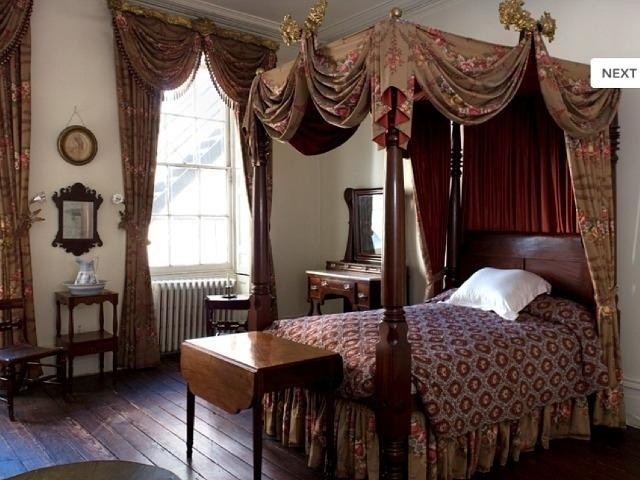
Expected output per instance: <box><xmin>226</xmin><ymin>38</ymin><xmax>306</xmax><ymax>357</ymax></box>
<box><xmin>0</xmin><ymin>298</ymin><xmax>63</xmax><ymax>422</ymax></box>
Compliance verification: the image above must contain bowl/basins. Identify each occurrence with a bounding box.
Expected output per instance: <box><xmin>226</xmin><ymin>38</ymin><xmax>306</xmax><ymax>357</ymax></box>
<box><xmin>63</xmin><ymin>279</ymin><xmax>108</xmax><ymax>295</ymax></box>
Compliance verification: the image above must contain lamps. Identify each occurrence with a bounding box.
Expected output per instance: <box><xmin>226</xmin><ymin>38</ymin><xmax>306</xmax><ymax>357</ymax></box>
<box><xmin>111</xmin><ymin>193</ymin><xmax>128</xmax><ymax>229</ymax></box>
<box><xmin>26</xmin><ymin>191</ymin><xmax>47</xmax><ymax>224</ymax></box>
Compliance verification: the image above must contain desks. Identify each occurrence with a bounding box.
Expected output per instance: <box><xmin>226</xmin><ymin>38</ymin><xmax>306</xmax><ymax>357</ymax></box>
<box><xmin>180</xmin><ymin>331</ymin><xmax>339</xmax><ymax>480</ymax></box>
<box><xmin>304</xmin><ymin>259</ymin><xmax>411</xmax><ymax>315</ymax></box>
<box><xmin>204</xmin><ymin>295</ymin><xmax>250</xmax><ymax>337</ymax></box>
<box><xmin>8</xmin><ymin>460</ymin><xmax>181</xmax><ymax>480</ymax></box>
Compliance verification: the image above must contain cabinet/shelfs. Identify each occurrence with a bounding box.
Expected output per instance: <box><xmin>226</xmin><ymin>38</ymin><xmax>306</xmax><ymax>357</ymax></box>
<box><xmin>54</xmin><ymin>290</ymin><xmax>119</xmax><ymax>389</ymax></box>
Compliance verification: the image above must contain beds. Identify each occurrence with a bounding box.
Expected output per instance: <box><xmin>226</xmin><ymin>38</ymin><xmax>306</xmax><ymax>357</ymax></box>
<box><xmin>243</xmin><ymin>0</ymin><xmax>626</xmax><ymax>480</ymax></box>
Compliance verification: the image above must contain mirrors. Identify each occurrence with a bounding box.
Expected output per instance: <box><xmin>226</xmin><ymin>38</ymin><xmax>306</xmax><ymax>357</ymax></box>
<box><xmin>344</xmin><ymin>187</ymin><xmax>384</xmax><ymax>265</ymax></box>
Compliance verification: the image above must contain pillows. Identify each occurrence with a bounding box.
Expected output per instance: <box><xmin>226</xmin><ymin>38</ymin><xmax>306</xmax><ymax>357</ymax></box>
<box><xmin>446</xmin><ymin>266</ymin><xmax>552</xmax><ymax>321</ymax></box>
<box><xmin>426</xmin><ymin>284</ymin><xmax>596</xmax><ymax>330</ymax></box>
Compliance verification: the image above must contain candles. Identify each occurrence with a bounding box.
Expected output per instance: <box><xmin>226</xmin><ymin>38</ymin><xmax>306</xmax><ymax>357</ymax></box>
<box><xmin>227</xmin><ymin>272</ymin><xmax>230</xmax><ymax>286</ymax></box>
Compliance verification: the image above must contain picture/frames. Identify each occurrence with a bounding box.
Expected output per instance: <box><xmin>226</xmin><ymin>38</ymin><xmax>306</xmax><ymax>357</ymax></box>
<box><xmin>51</xmin><ymin>182</ymin><xmax>103</xmax><ymax>256</ymax></box>
<box><xmin>57</xmin><ymin>125</ymin><xmax>98</xmax><ymax>165</ymax></box>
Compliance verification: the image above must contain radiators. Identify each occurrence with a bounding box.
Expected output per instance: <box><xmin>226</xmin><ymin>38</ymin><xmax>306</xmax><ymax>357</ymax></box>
<box><xmin>151</xmin><ymin>278</ymin><xmax>238</xmax><ymax>357</ymax></box>
